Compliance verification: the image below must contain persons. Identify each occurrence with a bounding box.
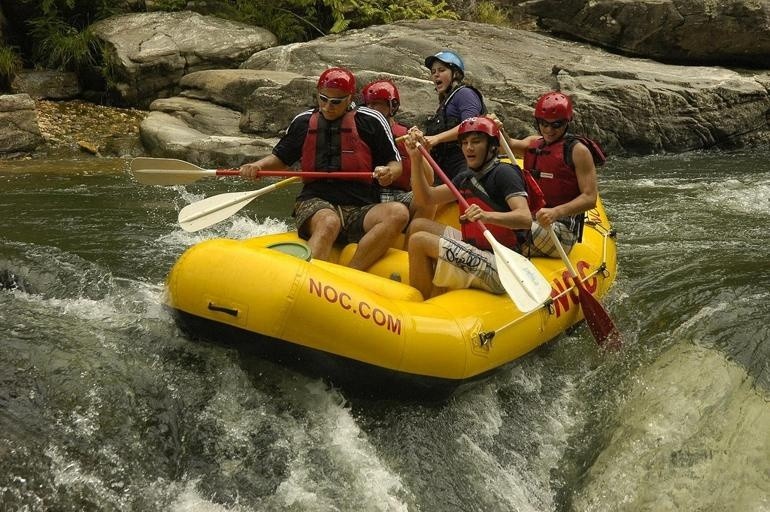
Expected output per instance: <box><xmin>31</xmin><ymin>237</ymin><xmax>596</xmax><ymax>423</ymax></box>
<box><xmin>488</xmin><ymin>93</ymin><xmax>606</xmax><ymax>259</ymax></box>
<box><xmin>406</xmin><ymin>52</ymin><xmax>487</xmax><ymax>188</ymax></box>
<box><xmin>407</xmin><ymin>117</ymin><xmax>532</xmax><ymax>302</ymax></box>
<box><xmin>360</xmin><ymin>80</ymin><xmax>414</xmax><ymax>205</ymax></box>
<box><xmin>238</xmin><ymin>67</ymin><xmax>410</xmax><ymax>272</ymax></box>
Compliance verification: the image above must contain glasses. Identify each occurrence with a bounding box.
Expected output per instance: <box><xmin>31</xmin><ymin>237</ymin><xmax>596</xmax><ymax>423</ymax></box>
<box><xmin>318</xmin><ymin>93</ymin><xmax>348</xmax><ymax>104</ymax></box>
<box><xmin>538</xmin><ymin>120</ymin><xmax>564</xmax><ymax>128</ymax></box>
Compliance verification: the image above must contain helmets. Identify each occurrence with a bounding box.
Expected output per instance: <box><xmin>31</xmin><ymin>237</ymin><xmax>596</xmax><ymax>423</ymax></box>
<box><xmin>362</xmin><ymin>80</ymin><xmax>399</xmax><ymax>116</ymax></box>
<box><xmin>318</xmin><ymin>68</ymin><xmax>356</xmax><ymax>93</ymax></box>
<box><xmin>425</xmin><ymin>51</ymin><xmax>464</xmax><ymax>75</ymax></box>
<box><xmin>535</xmin><ymin>92</ymin><xmax>572</xmax><ymax>121</ymax></box>
<box><xmin>458</xmin><ymin>117</ymin><xmax>500</xmax><ymax>146</ymax></box>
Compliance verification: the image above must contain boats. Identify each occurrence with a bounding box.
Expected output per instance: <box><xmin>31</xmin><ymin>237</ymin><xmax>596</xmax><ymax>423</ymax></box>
<box><xmin>164</xmin><ymin>157</ymin><xmax>610</xmax><ymax>396</ymax></box>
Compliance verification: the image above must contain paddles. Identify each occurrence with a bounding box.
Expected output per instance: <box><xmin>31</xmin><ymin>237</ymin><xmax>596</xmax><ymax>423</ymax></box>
<box><xmin>177</xmin><ymin>126</ymin><xmax>418</xmax><ymax>232</ymax></box>
<box><xmin>498</xmin><ymin>129</ymin><xmax>619</xmax><ymax>347</ymax></box>
<box><xmin>130</xmin><ymin>157</ymin><xmax>387</xmax><ymax>185</ymax></box>
<box><xmin>414</xmin><ymin>142</ymin><xmax>553</xmax><ymax>312</ymax></box>
